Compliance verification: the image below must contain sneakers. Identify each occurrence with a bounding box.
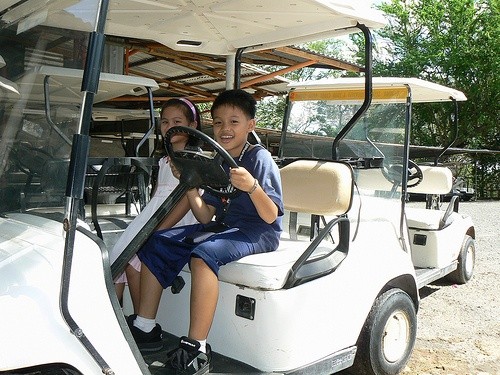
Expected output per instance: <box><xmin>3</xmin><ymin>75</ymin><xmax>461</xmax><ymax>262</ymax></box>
<box><xmin>148</xmin><ymin>336</ymin><xmax>213</xmax><ymax>375</ymax></box>
<box><xmin>129</xmin><ymin>323</ymin><xmax>164</xmax><ymax>352</ymax></box>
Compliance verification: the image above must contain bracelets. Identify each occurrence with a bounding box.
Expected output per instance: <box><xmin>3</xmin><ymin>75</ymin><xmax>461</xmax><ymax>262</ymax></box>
<box><xmin>248</xmin><ymin>179</ymin><xmax>259</xmax><ymax>195</ymax></box>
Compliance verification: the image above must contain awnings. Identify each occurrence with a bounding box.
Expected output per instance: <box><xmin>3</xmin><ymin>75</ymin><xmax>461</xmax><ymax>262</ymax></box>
<box><xmin>126</xmin><ymin>39</ymin><xmax>367</xmax><ymax>100</ymax></box>
<box><xmin>200</xmin><ymin>115</ymin><xmax>500</xmax><ymax>157</ymax></box>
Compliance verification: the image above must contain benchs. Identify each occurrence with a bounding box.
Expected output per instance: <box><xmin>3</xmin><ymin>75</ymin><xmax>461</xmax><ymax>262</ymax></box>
<box><xmin>181</xmin><ymin>158</ymin><xmax>460</xmax><ymax>290</ymax></box>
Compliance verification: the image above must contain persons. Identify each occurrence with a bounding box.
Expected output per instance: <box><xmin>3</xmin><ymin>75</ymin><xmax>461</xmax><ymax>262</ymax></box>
<box><xmin>109</xmin><ymin>96</ymin><xmax>208</xmax><ymax>327</ymax></box>
<box><xmin>130</xmin><ymin>90</ymin><xmax>285</xmax><ymax>375</ymax></box>
